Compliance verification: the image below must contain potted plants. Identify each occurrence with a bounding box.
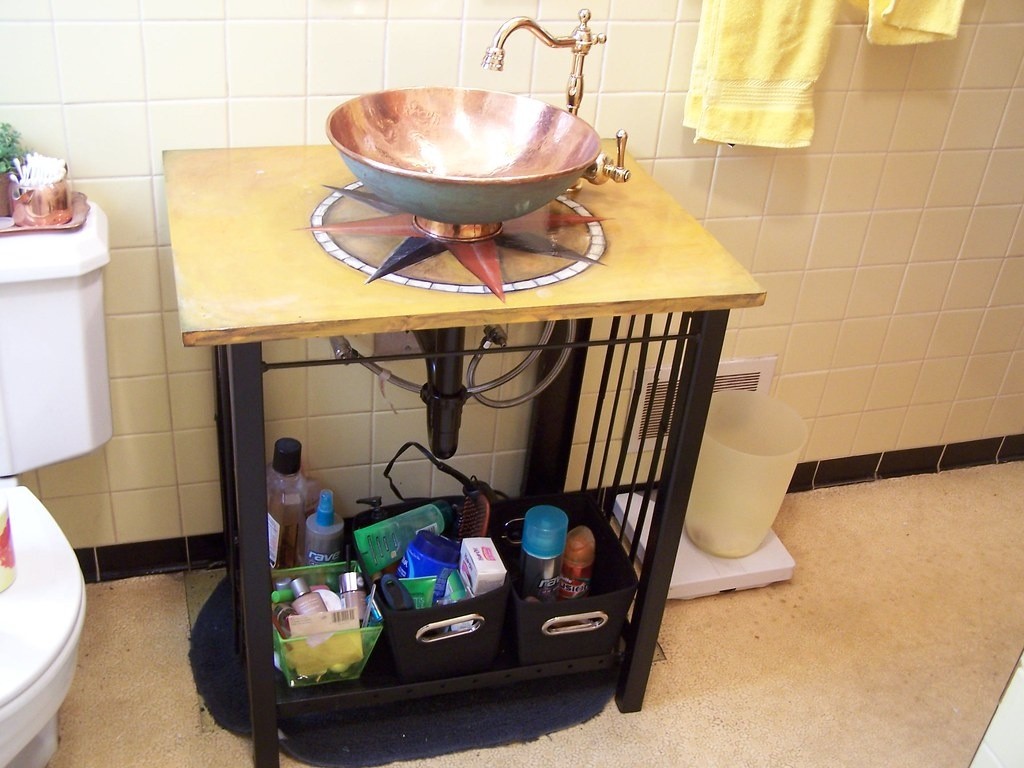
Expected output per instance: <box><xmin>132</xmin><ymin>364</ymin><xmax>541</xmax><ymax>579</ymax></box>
<box><xmin>0</xmin><ymin>122</ymin><xmax>24</xmax><ymax>217</ymax></box>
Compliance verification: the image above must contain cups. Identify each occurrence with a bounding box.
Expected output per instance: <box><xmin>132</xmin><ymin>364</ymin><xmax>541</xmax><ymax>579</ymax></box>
<box><xmin>0</xmin><ymin>499</ymin><xmax>17</xmax><ymax>592</ymax></box>
<box><xmin>8</xmin><ymin>160</ymin><xmax>72</xmax><ymax>228</ymax></box>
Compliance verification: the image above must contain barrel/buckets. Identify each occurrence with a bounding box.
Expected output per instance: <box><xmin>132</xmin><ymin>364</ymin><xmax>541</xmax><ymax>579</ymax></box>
<box><xmin>684</xmin><ymin>389</ymin><xmax>807</xmax><ymax>559</ymax></box>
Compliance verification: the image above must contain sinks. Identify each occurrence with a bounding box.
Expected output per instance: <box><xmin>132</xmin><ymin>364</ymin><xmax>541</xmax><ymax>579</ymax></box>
<box><xmin>324</xmin><ymin>83</ymin><xmax>604</xmax><ymax>225</ymax></box>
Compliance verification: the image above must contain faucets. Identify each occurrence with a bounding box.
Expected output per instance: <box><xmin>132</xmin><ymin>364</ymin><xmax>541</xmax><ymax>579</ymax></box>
<box><xmin>479</xmin><ymin>7</ymin><xmax>572</xmax><ymax>73</ymax></box>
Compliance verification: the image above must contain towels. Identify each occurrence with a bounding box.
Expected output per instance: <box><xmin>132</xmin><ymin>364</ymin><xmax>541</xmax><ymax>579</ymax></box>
<box><xmin>865</xmin><ymin>0</ymin><xmax>966</xmax><ymax>47</ymax></box>
<box><xmin>680</xmin><ymin>0</ymin><xmax>841</xmax><ymax>148</ymax></box>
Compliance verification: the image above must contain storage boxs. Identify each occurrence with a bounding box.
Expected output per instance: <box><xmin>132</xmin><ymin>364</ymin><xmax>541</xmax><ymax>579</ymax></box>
<box><xmin>351</xmin><ymin>504</ymin><xmax>512</xmax><ymax>678</ymax></box>
<box><xmin>272</xmin><ymin>560</ymin><xmax>383</xmax><ymax>688</ymax></box>
<box><xmin>459</xmin><ymin>536</ymin><xmax>507</xmax><ymax>599</ymax></box>
<box><xmin>492</xmin><ymin>494</ymin><xmax>639</xmax><ymax>665</ymax></box>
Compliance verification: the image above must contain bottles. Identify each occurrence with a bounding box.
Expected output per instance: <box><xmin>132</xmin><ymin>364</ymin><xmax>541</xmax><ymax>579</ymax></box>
<box><xmin>518</xmin><ymin>501</ymin><xmax>595</xmax><ymax>600</ymax></box>
<box><xmin>266</xmin><ymin>437</ymin><xmax>307</xmax><ymax>570</ymax></box>
<box><xmin>271</xmin><ymin>571</ymin><xmax>367</xmax><ymax>627</ymax></box>
<box><xmin>305</xmin><ymin>488</ymin><xmax>344</xmax><ymax>565</ymax></box>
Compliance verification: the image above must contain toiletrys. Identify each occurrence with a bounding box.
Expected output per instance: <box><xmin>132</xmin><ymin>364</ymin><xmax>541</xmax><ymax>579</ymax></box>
<box><xmin>266</xmin><ymin>436</ymin><xmax>507</xmax><ymax>639</ymax></box>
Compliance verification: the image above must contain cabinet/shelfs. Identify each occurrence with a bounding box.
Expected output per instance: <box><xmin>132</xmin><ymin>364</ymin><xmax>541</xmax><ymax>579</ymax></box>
<box><xmin>157</xmin><ymin>138</ymin><xmax>767</xmax><ymax>768</ymax></box>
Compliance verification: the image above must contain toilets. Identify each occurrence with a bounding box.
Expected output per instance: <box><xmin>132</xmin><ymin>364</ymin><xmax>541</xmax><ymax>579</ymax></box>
<box><xmin>0</xmin><ymin>193</ymin><xmax>115</xmax><ymax>768</ymax></box>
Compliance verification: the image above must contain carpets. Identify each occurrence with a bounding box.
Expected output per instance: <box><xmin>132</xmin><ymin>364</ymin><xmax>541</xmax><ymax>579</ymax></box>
<box><xmin>188</xmin><ymin>573</ymin><xmax>618</xmax><ymax>768</ymax></box>
